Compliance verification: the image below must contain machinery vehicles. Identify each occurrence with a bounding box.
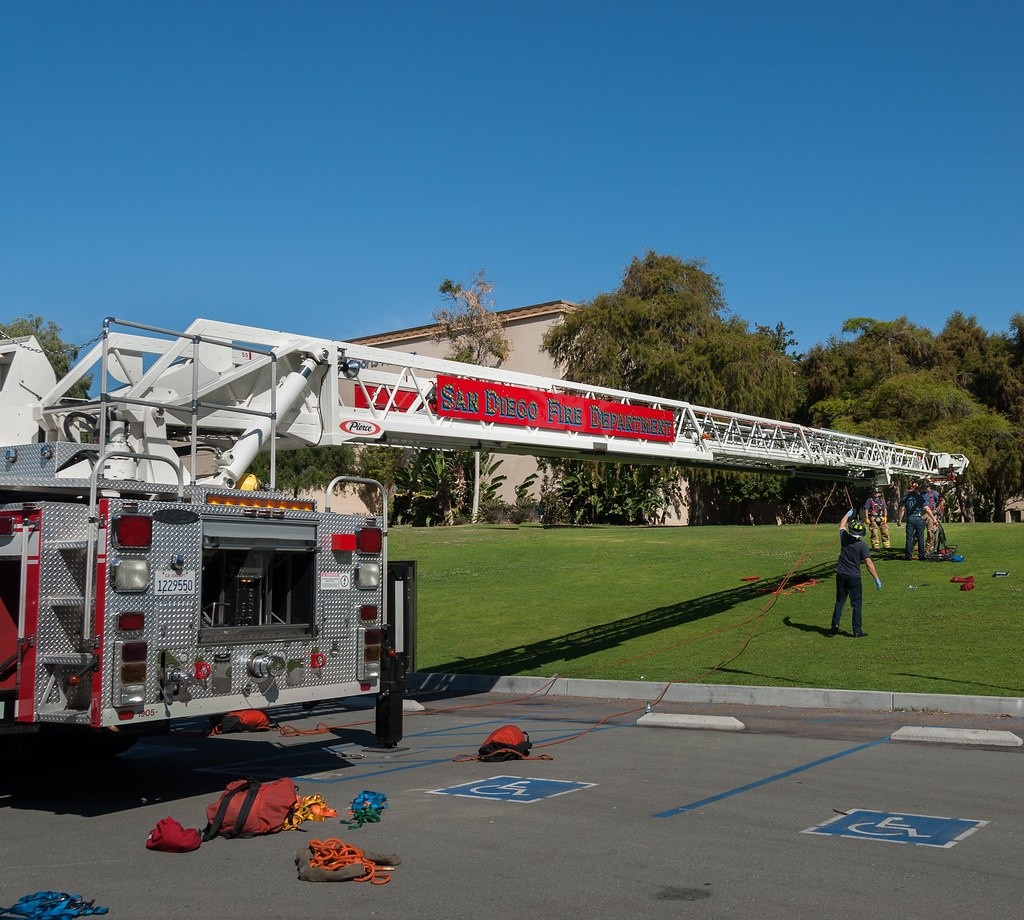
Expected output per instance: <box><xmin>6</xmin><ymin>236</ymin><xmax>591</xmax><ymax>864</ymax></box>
<box><xmin>1</xmin><ymin>318</ymin><xmax>973</xmax><ymax>751</ymax></box>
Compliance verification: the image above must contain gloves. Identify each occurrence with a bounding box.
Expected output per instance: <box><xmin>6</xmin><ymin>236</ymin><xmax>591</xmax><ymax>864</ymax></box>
<box><xmin>875</xmin><ymin>579</ymin><xmax>881</xmax><ymax>590</ymax></box>
<box><xmin>847</xmin><ymin>509</ymin><xmax>853</xmax><ymax>516</ymax></box>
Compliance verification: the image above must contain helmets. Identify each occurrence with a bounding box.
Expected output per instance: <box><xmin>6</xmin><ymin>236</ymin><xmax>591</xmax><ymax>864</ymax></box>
<box><xmin>849</xmin><ymin>519</ymin><xmax>866</xmax><ymax>538</ymax></box>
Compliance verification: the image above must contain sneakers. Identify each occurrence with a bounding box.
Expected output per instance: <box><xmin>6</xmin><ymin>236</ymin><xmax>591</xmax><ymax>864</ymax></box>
<box><xmin>855</xmin><ymin>633</ymin><xmax>868</xmax><ymax>638</ymax></box>
<box><xmin>829</xmin><ymin>628</ymin><xmax>839</xmax><ymax>637</ymax></box>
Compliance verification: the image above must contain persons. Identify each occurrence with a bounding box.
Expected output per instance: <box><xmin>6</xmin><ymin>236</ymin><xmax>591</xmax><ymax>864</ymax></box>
<box><xmin>867</xmin><ymin>490</ymin><xmax>893</xmax><ymax>552</ymax></box>
<box><xmin>896</xmin><ymin>483</ymin><xmax>938</xmax><ymax>561</ymax></box>
<box><xmin>911</xmin><ymin>482</ymin><xmax>944</xmax><ymax>554</ymax></box>
<box><xmin>827</xmin><ymin>508</ymin><xmax>882</xmax><ymax>638</ymax></box>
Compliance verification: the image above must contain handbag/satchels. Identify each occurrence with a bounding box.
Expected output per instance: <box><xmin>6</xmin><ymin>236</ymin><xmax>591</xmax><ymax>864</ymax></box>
<box><xmin>201</xmin><ymin>776</ymin><xmax>298</xmax><ymax>842</ymax></box>
